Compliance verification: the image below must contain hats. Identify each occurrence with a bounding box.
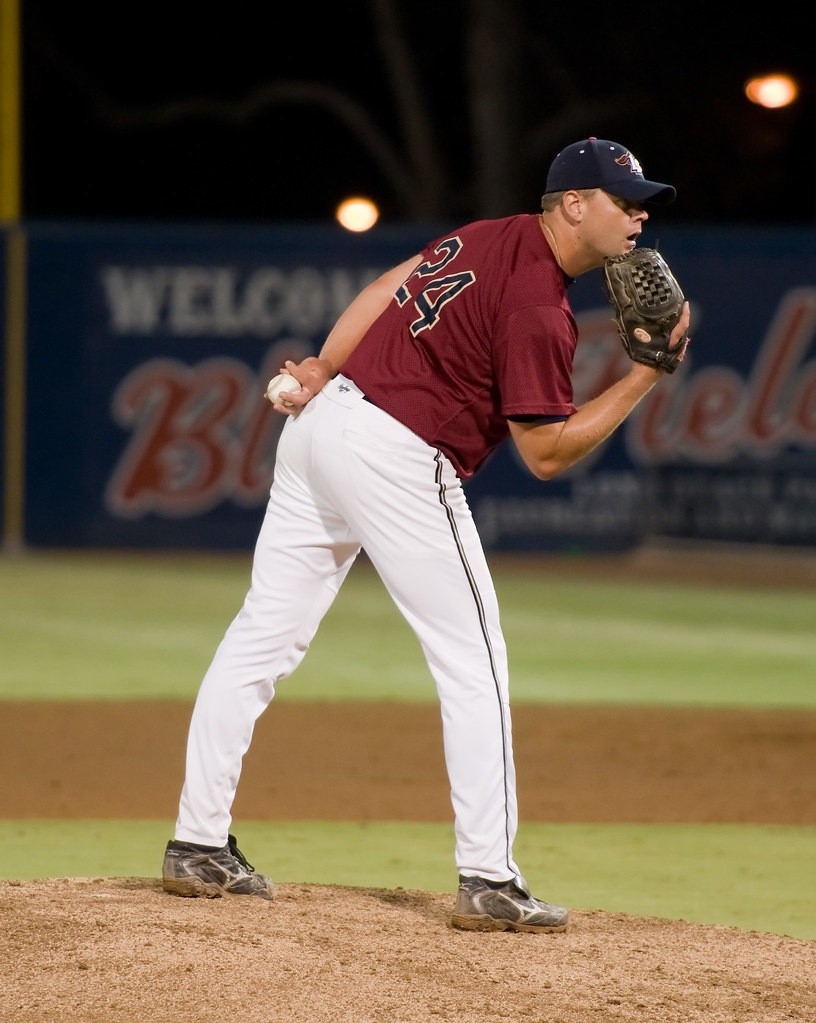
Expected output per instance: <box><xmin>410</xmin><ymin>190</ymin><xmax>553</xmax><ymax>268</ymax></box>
<box><xmin>545</xmin><ymin>137</ymin><xmax>676</xmax><ymax>208</ymax></box>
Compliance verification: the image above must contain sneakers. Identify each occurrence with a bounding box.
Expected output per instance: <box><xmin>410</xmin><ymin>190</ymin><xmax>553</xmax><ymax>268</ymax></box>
<box><xmin>160</xmin><ymin>833</ymin><xmax>274</xmax><ymax>897</ymax></box>
<box><xmin>450</xmin><ymin>874</ymin><xmax>569</xmax><ymax>933</ymax></box>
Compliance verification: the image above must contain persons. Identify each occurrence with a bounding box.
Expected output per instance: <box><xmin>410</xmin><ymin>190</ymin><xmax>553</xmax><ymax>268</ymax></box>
<box><xmin>161</xmin><ymin>137</ymin><xmax>690</xmax><ymax>932</ymax></box>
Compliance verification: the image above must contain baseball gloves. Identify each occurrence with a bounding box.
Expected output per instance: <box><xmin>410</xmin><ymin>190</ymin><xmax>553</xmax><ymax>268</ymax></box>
<box><xmin>604</xmin><ymin>248</ymin><xmax>689</xmax><ymax>374</ymax></box>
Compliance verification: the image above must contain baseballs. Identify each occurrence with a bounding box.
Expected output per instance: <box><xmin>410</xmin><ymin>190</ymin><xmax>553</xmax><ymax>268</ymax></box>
<box><xmin>267</xmin><ymin>374</ymin><xmax>302</xmax><ymax>407</ymax></box>
